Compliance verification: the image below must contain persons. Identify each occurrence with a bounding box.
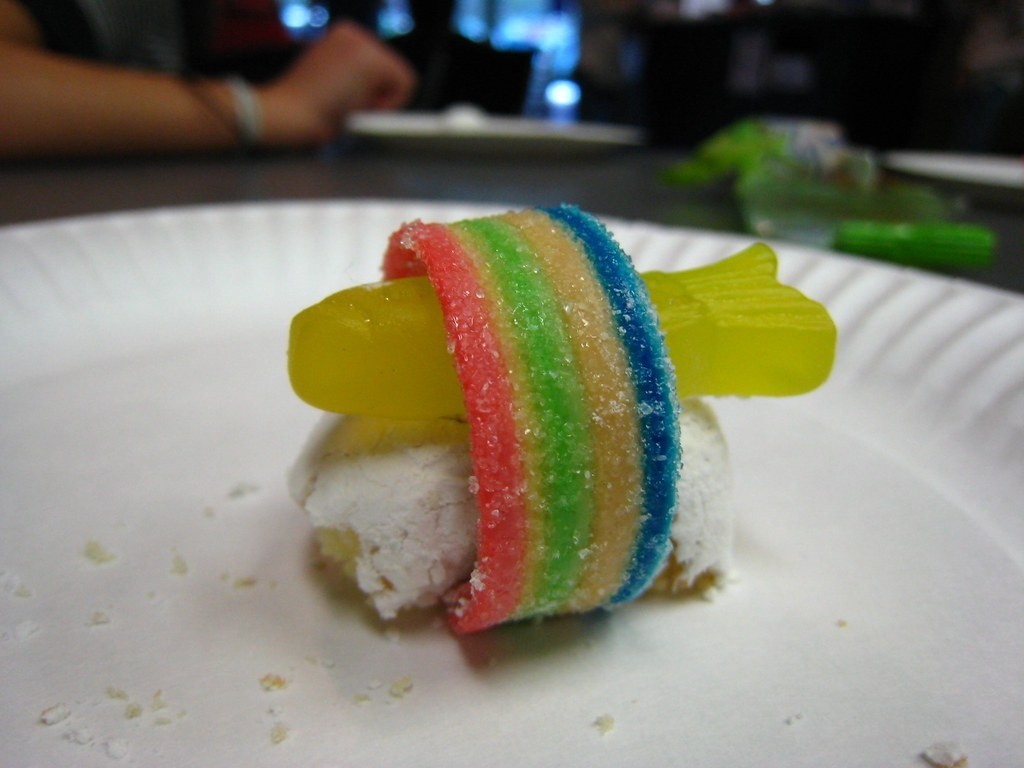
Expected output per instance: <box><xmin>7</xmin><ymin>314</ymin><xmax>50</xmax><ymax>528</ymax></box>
<box><xmin>0</xmin><ymin>0</ymin><xmax>417</xmax><ymax>163</ymax></box>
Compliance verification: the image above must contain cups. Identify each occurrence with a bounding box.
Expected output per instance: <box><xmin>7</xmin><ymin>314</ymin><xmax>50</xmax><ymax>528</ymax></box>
<box><xmin>733</xmin><ymin>112</ymin><xmax>875</xmax><ymax>244</ymax></box>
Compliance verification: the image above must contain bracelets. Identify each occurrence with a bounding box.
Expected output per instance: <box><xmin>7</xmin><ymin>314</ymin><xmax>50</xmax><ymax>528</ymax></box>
<box><xmin>231</xmin><ymin>76</ymin><xmax>266</xmax><ymax>158</ymax></box>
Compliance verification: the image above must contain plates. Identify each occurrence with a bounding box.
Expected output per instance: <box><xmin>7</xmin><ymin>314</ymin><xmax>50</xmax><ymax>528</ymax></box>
<box><xmin>347</xmin><ymin>106</ymin><xmax>641</xmax><ymax>157</ymax></box>
<box><xmin>0</xmin><ymin>197</ymin><xmax>1024</xmax><ymax>768</ymax></box>
<box><xmin>885</xmin><ymin>147</ymin><xmax>1024</xmax><ymax>192</ymax></box>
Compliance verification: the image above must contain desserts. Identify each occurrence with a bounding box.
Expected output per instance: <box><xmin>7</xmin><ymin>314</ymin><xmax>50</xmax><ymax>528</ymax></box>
<box><xmin>285</xmin><ymin>199</ymin><xmax>735</xmax><ymax>607</ymax></box>
